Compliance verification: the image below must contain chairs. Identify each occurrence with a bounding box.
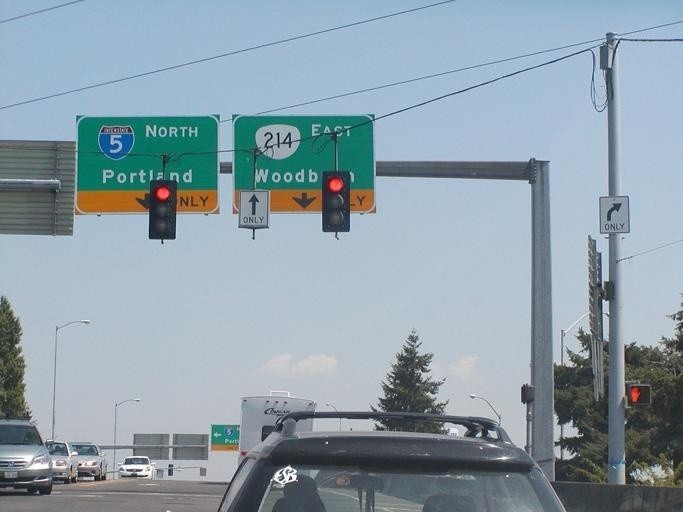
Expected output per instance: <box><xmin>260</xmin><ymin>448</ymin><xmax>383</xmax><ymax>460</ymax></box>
<box><xmin>422</xmin><ymin>495</ymin><xmax>477</xmax><ymax>512</ymax></box>
<box><xmin>272</xmin><ymin>475</ymin><xmax>326</xmax><ymax>512</ymax></box>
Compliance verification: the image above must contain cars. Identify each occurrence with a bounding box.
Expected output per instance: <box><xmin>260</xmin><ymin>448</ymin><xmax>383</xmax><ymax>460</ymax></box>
<box><xmin>68</xmin><ymin>442</ymin><xmax>107</xmax><ymax>481</ymax></box>
<box><xmin>0</xmin><ymin>419</ymin><xmax>53</xmax><ymax>495</ymax></box>
<box><xmin>118</xmin><ymin>456</ymin><xmax>154</xmax><ymax>480</ymax></box>
<box><xmin>45</xmin><ymin>442</ymin><xmax>77</xmax><ymax>484</ymax></box>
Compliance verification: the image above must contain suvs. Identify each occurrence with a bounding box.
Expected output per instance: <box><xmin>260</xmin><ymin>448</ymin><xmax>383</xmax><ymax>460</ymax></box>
<box><xmin>215</xmin><ymin>410</ymin><xmax>568</xmax><ymax>512</ymax></box>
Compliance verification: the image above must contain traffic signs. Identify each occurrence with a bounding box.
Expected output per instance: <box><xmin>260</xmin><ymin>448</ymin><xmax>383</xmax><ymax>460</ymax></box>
<box><xmin>231</xmin><ymin>114</ymin><xmax>377</xmax><ymax>215</ymax></box>
<box><xmin>210</xmin><ymin>424</ymin><xmax>241</xmax><ymax>450</ymax></box>
<box><xmin>74</xmin><ymin>116</ymin><xmax>218</xmax><ymax>215</ymax></box>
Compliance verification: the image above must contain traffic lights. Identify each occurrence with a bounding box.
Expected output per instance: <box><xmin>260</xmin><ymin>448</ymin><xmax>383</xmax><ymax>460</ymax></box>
<box><xmin>628</xmin><ymin>384</ymin><xmax>652</xmax><ymax>406</ymax></box>
<box><xmin>321</xmin><ymin>170</ymin><xmax>350</xmax><ymax>234</ymax></box>
<box><xmin>149</xmin><ymin>179</ymin><xmax>178</xmax><ymax>241</ymax></box>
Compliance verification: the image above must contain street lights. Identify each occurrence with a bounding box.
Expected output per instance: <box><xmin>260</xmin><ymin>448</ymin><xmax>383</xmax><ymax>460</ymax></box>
<box><xmin>113</xmin><ymin>398</ymin><xmax>139</xmax><ymax>478</ymax></box>
<box><xmin>326</xmin><ymin>404</ymin><xmax>341</xmax><ymax>431</ymax></box>
<box><xmin>559</xmin><ymin>312</ymin><xmax>609</xmax><ymax>459</ymax></box>
<box><xmin>470</xmin><ymin>395</ymin><xmax>502</xmax><ymax>426</ymax></box>
<box><xmin>51</xmin><ymin>321</ymin><xmax>90</xmax><ymax>440</ymax></box>
